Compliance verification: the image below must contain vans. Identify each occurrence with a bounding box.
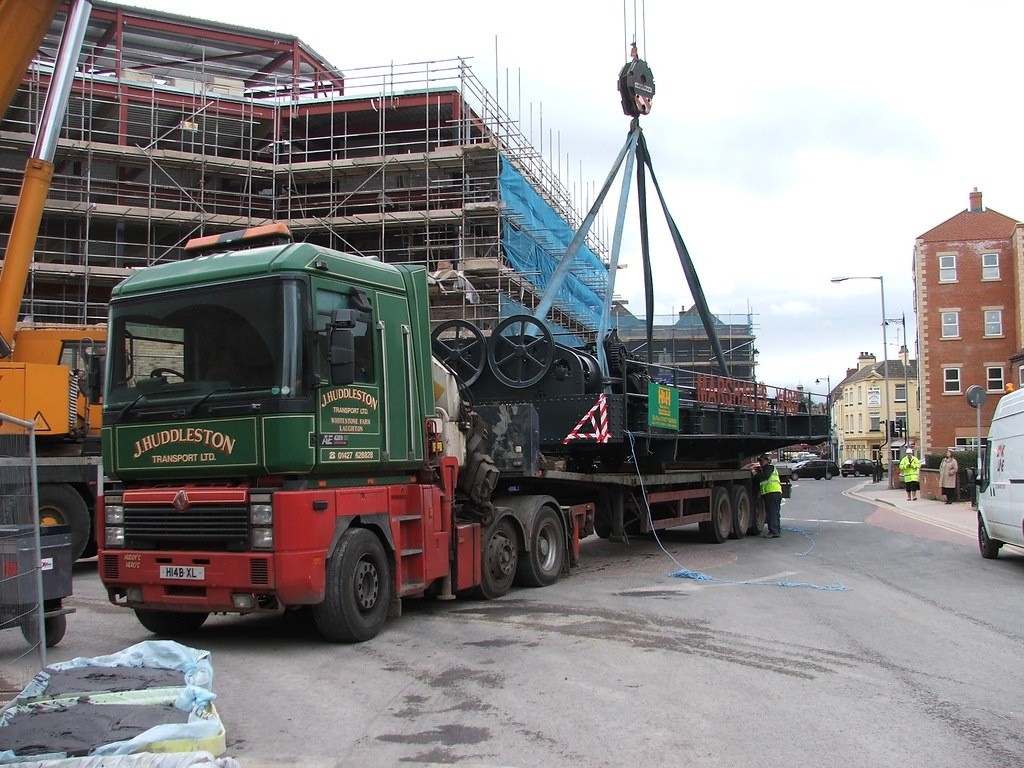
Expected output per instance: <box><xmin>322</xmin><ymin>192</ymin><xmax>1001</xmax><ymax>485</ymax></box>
<box><xmin>963</xmin><ymin>384</ymin><xmax>1024</xmax><ymax>559</ymax></box>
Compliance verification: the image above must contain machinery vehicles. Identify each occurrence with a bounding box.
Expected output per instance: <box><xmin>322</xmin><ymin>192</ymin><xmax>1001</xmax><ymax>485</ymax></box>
<box><xmin>0</xmin><ymin>0</ymin><xmax>116</xmax><ymax>563</ymax></box>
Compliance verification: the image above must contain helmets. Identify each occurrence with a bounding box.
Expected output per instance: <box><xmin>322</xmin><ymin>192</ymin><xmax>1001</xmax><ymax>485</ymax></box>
<box><xmin>906</xmin><ymin>448</ymin><xmax>914</xmax><ymax>454</ymax></box>
<box><xmin>757</xmin><ymin>454</ymin><xmax>773</xmax><ymax>463</ymax></box>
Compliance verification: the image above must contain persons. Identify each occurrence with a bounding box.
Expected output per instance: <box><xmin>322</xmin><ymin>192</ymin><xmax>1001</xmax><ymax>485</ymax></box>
<box><xmin>899</xmin><ymin>448</ymin><xmax>921</xmax><ymax>501</ymax></box>
<box><xmin>939</xmin><ymin>450</ymin><xmax>958</xmax><ymax>504</ymax></box>
<box><xmin>752</xmin><ymin>454</ymin><xmax>782</xmax><ymax>538</ymax></box>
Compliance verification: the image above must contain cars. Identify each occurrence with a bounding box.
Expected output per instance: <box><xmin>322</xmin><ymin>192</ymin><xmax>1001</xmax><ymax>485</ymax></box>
<box><xmin>776</xmin><ymin>452</ymin><xmax>823</xmax><ymax>472</ymax></box>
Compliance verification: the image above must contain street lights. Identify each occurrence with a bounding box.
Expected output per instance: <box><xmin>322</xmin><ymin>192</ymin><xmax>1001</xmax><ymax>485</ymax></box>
<box><xmin>831</xmin><ymin>275</ymin><xmax>891</xmax><ymax>488</ymax></box>
<box><xmin>814</xmin><ymin>375</ymin><xmax>832</xmax><ymax>458</ymax></box>
<box><xmin>880</xmin><ymin>310</ymin><xmax>910</xmax><ymax>447</ymax></box>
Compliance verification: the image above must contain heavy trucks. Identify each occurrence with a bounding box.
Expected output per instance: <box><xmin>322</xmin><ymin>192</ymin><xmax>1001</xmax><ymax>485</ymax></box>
<box><xmin>75</xmin><ymin>220</ymin><xmax>794</xmax><ymax>645</ymax></box>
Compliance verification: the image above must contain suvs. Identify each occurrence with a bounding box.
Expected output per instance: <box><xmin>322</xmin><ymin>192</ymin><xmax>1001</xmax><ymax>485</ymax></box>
<box><xmin>842</xmin><ymin>459</ymin><xmax>883</xmax><ymax>477</ymax></box>
<box><xmin>790</xmin><ymin>460</ymin><xmax>841</xmax><ymax>481</ymax></box>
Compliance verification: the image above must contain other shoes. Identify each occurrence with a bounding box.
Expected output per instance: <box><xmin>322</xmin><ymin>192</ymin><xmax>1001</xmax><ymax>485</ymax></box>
<box><xmin>913</xmin><ymin>497</ymin><xmax>917</xmax><ymax>501</ymax></box>
<box><xmin>907</xmin><ymin>498</ymin><xmax>911</xmax><ymax>501</ymax></box>
<box><xmin>764</xmin><ymin>531</ymin><xmax>780</xmax><ymax>538</ymax></box>
<box><xmin>945</xmin><ymin>500</ymin><xmax>952</xmax><ymax>504</ymax></box>
